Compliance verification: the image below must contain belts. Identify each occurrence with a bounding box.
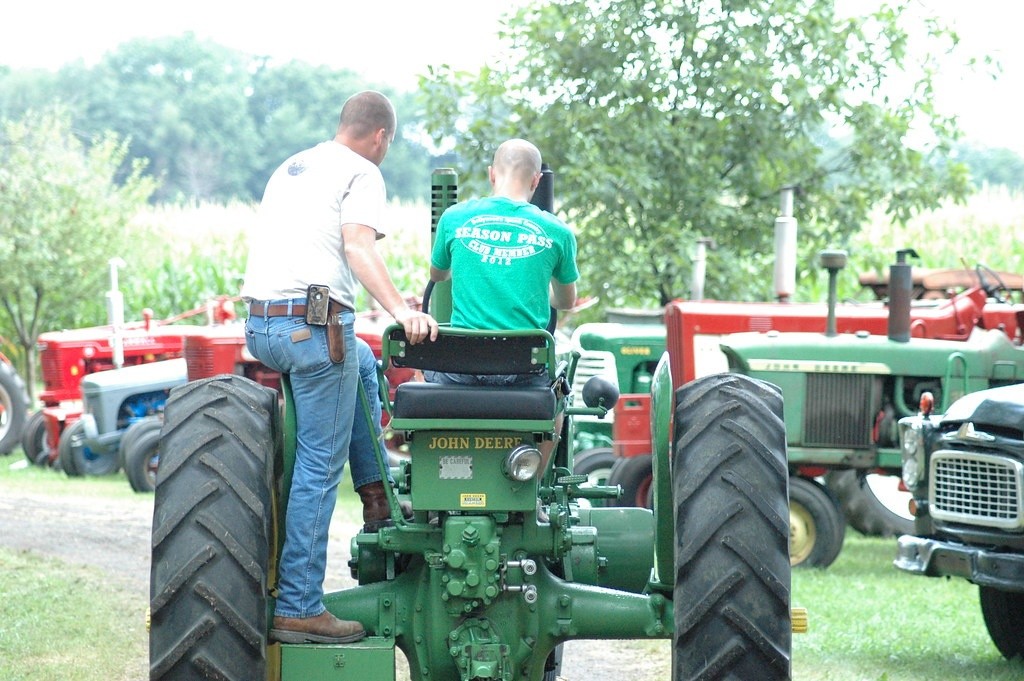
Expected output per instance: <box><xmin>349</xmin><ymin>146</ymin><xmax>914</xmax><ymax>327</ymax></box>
<box><xmin>250</xmin><ymin>301</ymin><xmax>349</xmax><ymax>317</ymax></box>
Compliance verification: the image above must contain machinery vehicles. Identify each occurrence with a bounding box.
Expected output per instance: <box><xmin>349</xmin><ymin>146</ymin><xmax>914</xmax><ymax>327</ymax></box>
<box><xmin>148</xmin><ymin>161</ymin><xmax>793</xmax><ymax>681</ymax></box>
<box><xmin>0</xmin><ymin>182</ymin><xmax>1024</xmax><ymax>657</ymax></box>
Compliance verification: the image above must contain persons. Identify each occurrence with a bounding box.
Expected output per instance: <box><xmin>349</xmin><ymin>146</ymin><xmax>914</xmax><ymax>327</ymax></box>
<box><xmin>242</xmin><ymin>90</ymin><xmax>439</xmax><ymax>644</ymax></box>
<box><xmin>415</xmin><ymin>138</ymin><xmax>580</xmax><ymax>522</ymax></box>
<box><xmin>234</xmin><ymin>279</ymin><xmax>253</xmax><ymax>316</ymax></box>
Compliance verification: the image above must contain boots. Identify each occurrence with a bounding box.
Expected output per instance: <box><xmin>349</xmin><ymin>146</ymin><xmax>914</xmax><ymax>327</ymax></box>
<box><xmin>359</xmin><ymin>482</ymin><xmax>414</xmax><ymax>532</ymax></box>
<box><xmin>270</xmin><ymin>609</ymin><xmax>367</xmax><ymax>645</ymax></box>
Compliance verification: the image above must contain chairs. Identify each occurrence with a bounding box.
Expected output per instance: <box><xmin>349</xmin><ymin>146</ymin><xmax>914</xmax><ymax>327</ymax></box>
<box><xmin>380</xmin><ymin>325</ymin><xmax>559</xmax><ymax>422</ymax></box>
